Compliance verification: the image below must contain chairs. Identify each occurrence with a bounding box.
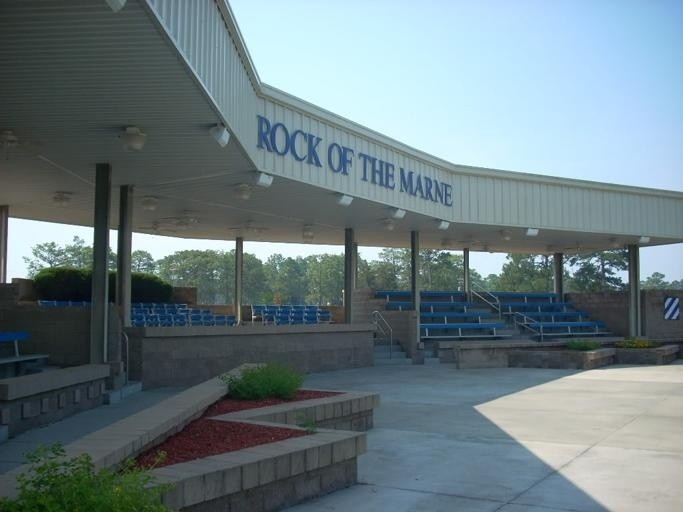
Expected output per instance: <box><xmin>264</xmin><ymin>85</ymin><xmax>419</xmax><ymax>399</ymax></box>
<box><xmin>41</xmin><ymin>298</ymin><xmax>335</xmax><ymax>328</ymax></box>
<box><xmin>0</xmin><ymin>329</ymin><xmax>31</xmax><ymax>357</ymax></box>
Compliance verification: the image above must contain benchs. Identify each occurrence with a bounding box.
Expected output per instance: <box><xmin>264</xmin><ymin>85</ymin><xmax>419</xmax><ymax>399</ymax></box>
<box><xmin>375</xmin><ymin>288</ymin><xmax>619</xmax><ymax>344</ymax></box>
